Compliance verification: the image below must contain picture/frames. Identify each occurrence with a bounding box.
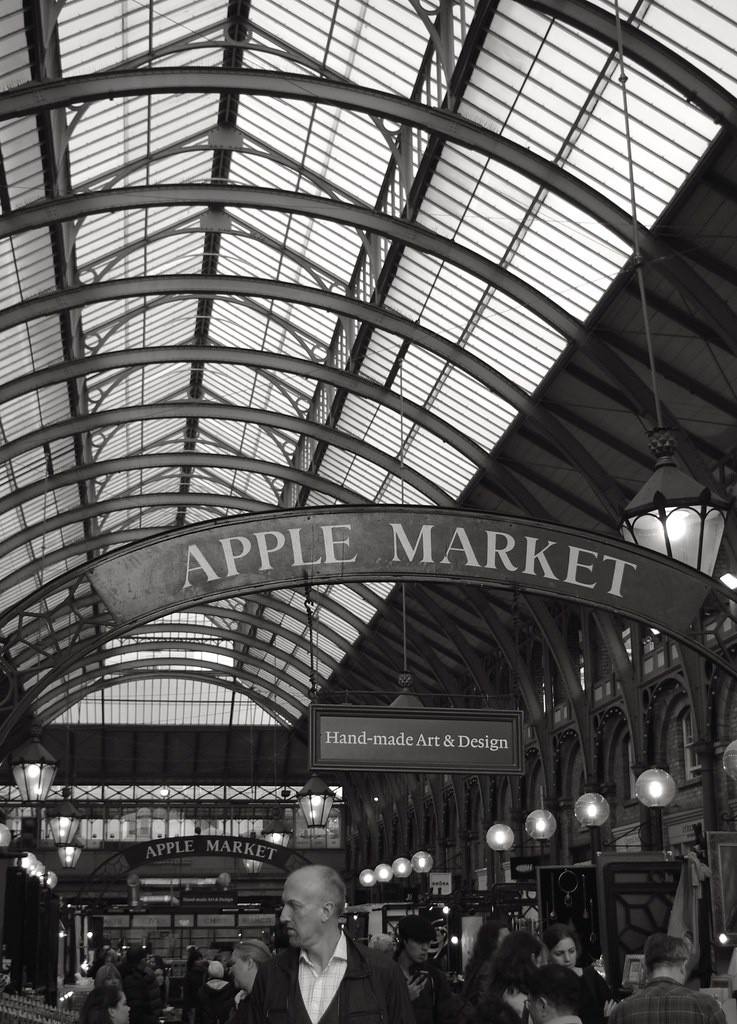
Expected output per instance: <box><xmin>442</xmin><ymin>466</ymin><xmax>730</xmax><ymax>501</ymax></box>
<box><xmin>706</xmin><ymin>830</ymin><xmax>737</xmax><ymax>947</ymax></box>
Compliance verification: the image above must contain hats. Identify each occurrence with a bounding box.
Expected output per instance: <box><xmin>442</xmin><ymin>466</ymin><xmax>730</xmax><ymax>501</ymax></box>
<box><xmin>399</xmin><ymin>914</ymin><xmax>436</xmax><ymax>941</ymax></box>
<box><xmin>127</xmin><ymin>943</ymin><xmax>146</xmax><ymax>962</ymax></box>
<box><xmin>208</xmin><ymin>960</ymin><xmax>224</xmax><ymax>979</ymax></box>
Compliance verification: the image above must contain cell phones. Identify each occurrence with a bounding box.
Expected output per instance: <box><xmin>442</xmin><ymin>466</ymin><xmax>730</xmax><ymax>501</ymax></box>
<box><xmin>410</xmin><ymin>971</ymin><xmax>428</xmax><ymax>986</ymax></box>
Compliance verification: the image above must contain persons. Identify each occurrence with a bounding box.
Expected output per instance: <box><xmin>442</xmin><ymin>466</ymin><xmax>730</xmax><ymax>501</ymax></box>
<box><xmin>81</xmin><ymin>865</ymin><xmax>727</xmax><ymax>1024</ymax></box>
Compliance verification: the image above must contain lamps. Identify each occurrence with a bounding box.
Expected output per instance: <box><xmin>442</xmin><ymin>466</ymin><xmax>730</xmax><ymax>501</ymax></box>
<box><xmin>246</xmin><ymin>785</ymin><xmax>264</xmax><ymax>877</ymax></box>
<box><xmin>262</xmin><ymin>635</ymin><xmax>294</xmax><ymax>847</ymax></box>
<box><xmin>575</xmin><ymin>793</ymin><xmax>612</xmax><ymax>854</ymax></box>
<box><xmin>634</xmin><ymin>768</ymin><xmax>677</xmax><ymax>850</ymax></box>
<box><xmin>359</xmin><ymin>848</ymin><xmax>434</xmax><ymax>897</ymax></box>
<box><xmin>614</xmin><ymin>0</ymin><xmax>737</xmax><ymax>581</ymax></box>
<box><xmin>10</xmin><ymin>453</ymin><xmax>61</xmax><ymax>806</ymax></box>
<box><xmin>388</xmin><ymin>359</ymin><xmax>426</xmax><ymax>708</ymax></box>
<box><xmin>296</xmin><ymin>584</ymin><xmax>335</xmax><ymax>827</ymax></box>
<box><xmin>485</xmin><ymin>824</ymin><xmax>514</xmax><ymax>863</ymax></box>
<box><xmin>14</xmin><ymin>852</ymin><xmax>57</xmax><ymax>889</ymax></box>
<box><xmin>57</xmin><ymin>838</ymin><xmax>84</xmax><ymax>869</ymax></box>
<box><xmin>526</xmin><ymin>809</ymin><xmax>556</xmax><ymax>855</ymax></box>
<box><xmin>46</xmin><ymin>673</ymin><xmax>83</xmax><ymax>846</ymax></box>
